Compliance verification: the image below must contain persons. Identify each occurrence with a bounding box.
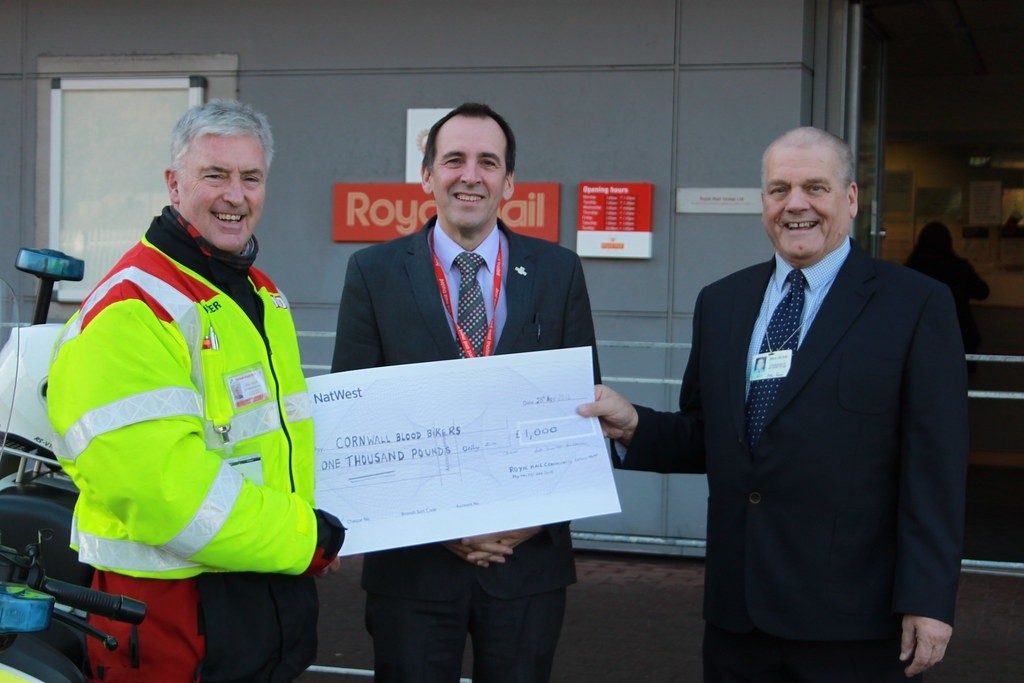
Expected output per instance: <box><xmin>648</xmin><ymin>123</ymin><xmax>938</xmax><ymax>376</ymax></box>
<box><xmin>51</xmin><ymin>95</ymin><xmax>351</xmax><ymax>683</ymax></box>
<box><xmin>332</xmin><ymin>102</ymin><xmax>602</xmax><ymax>683</ymax></box>
<box><xmin>899</xmin><ymin>220</ymin><xmax>994</xmax><ymax>353</ymax></box>
<box><xmin>576</xmin><ymin>125</ymin><xmax>967</xmax><ymax>683</ymax></box>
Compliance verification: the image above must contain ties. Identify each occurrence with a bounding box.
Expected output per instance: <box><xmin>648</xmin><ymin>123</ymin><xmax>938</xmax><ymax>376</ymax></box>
<box><xmin>744</xmin><ymin>269</ymin><xmax>808</xmax><ymax>455</ymax></box>
<box><xmin>454</xmin><ymin>252</ymin><xmax>491</xmax><ymax>359</ymax></box>
